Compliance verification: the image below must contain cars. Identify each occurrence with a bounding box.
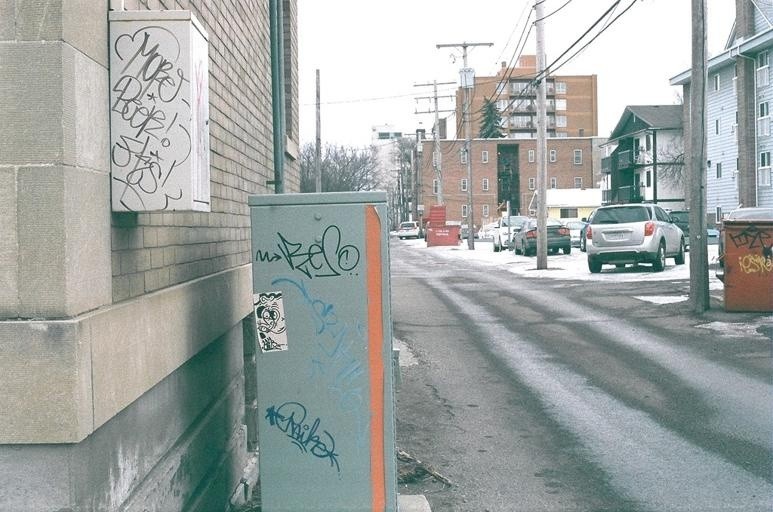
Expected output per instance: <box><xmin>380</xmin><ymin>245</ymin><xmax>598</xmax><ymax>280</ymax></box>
<box><xmin>478</xmin><ymin>200</ymin><xmax>720</xmax><ymax>273</ymax></box>
<box><xmin>397</xmin><ymin>221</ymin><xmax>421</xmax><ymax>240</ymax></box>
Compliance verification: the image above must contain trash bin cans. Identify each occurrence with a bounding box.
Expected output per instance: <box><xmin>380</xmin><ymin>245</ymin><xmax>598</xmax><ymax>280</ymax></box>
<box><xmin>721</xmin><ymin>207</ymin><xmax>773</xmax><ymax>312</ymax></box>
